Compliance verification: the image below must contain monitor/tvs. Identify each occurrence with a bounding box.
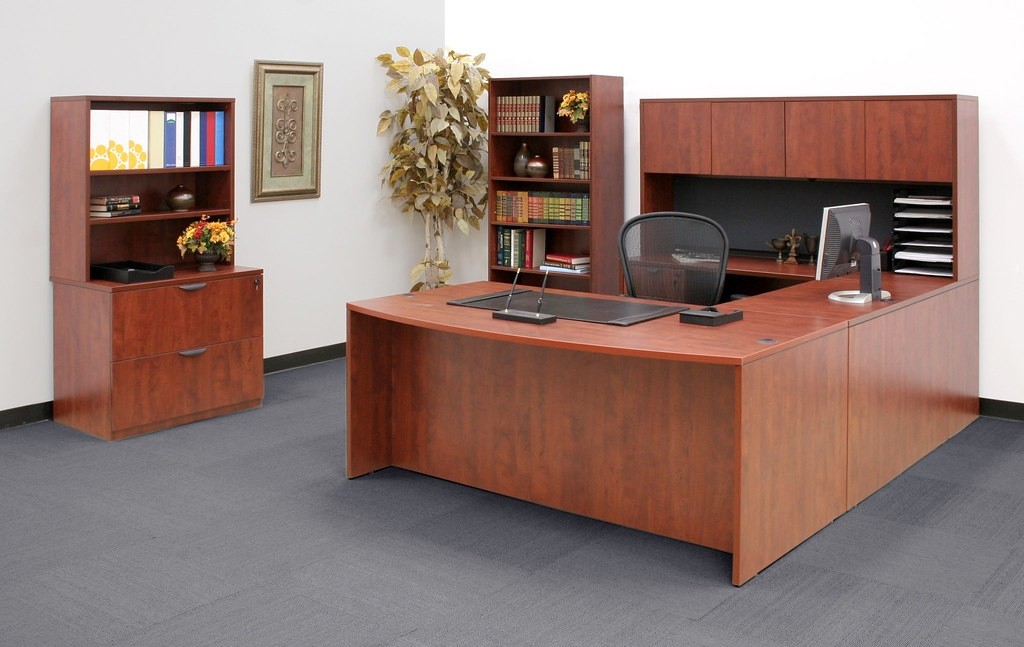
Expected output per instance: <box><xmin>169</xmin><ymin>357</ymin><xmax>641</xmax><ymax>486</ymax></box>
<box><xmin>816</xmin><ymin>203</ymin><xmax>892</xmax><ymax>303</ymax></box>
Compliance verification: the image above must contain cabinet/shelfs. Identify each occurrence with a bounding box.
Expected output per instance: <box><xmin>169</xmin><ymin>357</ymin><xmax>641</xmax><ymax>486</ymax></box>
<box><xmin>49</xmin><ymin>96</ymin><xmax>263</xmax><ymax>441</ymax></box>
<box><xmin>638</xmin><ymin>94</ymin><xmax>981</xmax><ymax>282</ymax></box>
<box><xmin>487</xmin><ymin>74</ymin><xmax>626</xmax><ymax>294</ymax></box>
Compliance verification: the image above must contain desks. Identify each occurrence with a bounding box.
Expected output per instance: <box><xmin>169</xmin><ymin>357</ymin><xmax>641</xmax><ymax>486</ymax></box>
<box><xmin>345</xmin><ymin>250</ymin><xmax>980</xmax><ymax>586</ymax></box>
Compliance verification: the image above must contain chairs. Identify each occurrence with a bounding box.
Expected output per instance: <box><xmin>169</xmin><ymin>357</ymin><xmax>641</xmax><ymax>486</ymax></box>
<box><xmin>617</xmin><ymin>212</ymin><xmax>728</xmax><ymax>306</ymax></box>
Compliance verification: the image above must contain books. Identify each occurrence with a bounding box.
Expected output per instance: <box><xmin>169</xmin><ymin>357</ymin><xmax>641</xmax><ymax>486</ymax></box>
<box><xmin>90</xmin><ymin>195</ymin><xmax>142</xmax><ymax>217</ymax></box>
<box><xmin>553</xmin><ymin>142</ymin><xmax>591</xmax><ymax>180</ymax></box>
<box><xmin>496</xmin><ymin>96</ymin><xmax>555</xmax><ymax>133</ymax></box>
<box><xmin>893</xmin><ymin>194</ymin><xmax>954</xmax><ymax>278</ymax></box>
<box><xmin>496</xmin><ymin>184</ymin><xmax>591</xmax><ymax>226</ymax></box>
<box><xmin>497</xmin><ymin>227</ymin><xmax>591</xmax><ymax>274</ymax></box>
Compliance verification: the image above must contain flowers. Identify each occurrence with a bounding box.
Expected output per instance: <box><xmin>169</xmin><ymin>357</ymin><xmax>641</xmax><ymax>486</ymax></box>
<box><xmin>556</xmin><ymin>90</ymin><xmax>590</xmax><ymax>124</ymax></box>
<box><xmin>177</xmin><ymin>214</ymin><xmax>235</xmax><ymax>262</ymax></box>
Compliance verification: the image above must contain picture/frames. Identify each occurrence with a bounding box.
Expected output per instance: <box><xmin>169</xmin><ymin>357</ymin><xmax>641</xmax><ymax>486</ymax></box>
<box><xmin>251</xmin><ymin>59</ymin><xmax>324</xmax><ymax>202</ymax></box>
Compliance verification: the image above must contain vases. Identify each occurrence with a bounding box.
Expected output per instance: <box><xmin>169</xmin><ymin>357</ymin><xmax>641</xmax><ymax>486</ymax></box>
<box><xmin>575</xmin><ymin>111</ymin><xmax>589</xmax><ymax>133</ymax></box>
<box><xmin>194</xmin><ymin>251</ymin><xmax>220</xmax><ymax>272</ymax></box>
